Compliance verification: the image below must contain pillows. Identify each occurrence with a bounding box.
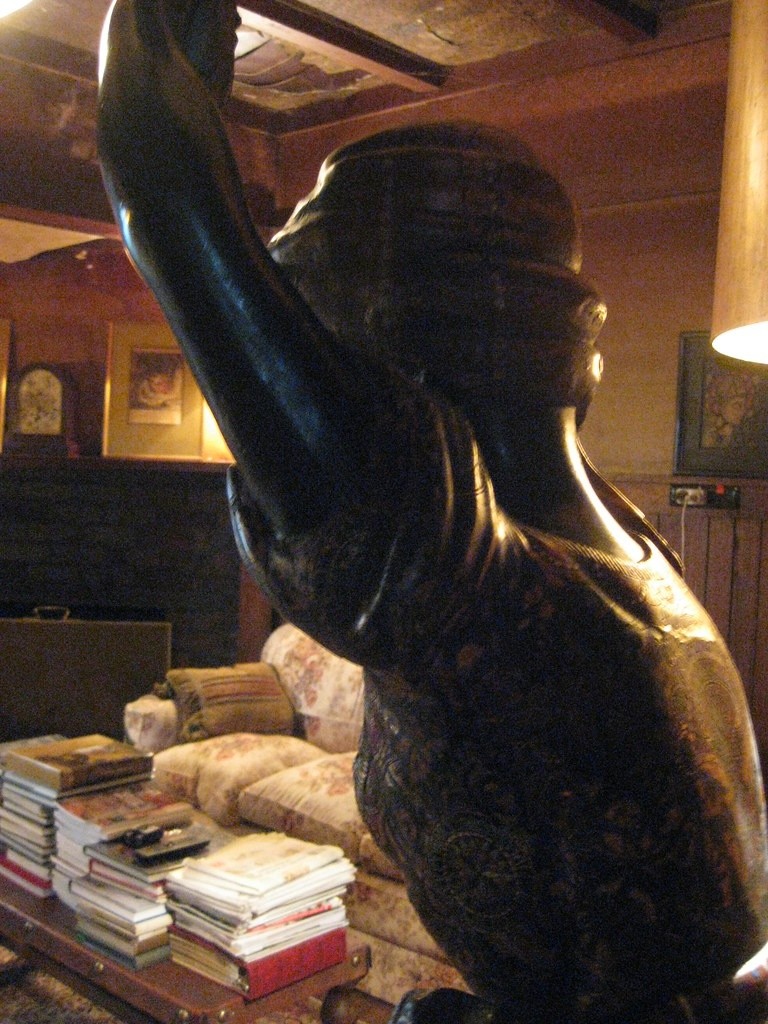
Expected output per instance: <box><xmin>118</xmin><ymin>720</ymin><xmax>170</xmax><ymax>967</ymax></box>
<box><xmin>165</xmin><ymin>661</ymin><xmax>296</xmax><ymax>742</ymax></box>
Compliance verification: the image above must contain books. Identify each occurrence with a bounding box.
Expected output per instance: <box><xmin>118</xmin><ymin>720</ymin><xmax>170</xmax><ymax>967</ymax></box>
<box><xmin>0</xmin><ymin>730</ymin><xmax>356</xmax><ymax>995</ymax></box>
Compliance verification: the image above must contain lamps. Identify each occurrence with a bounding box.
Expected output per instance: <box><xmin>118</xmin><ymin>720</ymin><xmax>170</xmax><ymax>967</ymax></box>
<box><xmin>710</xmin><ymin>0</ymin><xmax>768</xmax><ymax>367</ymax></box>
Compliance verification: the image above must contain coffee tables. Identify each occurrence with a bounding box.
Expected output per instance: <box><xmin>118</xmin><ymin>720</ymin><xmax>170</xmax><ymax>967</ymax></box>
<box><xmin>0</xmin><ymin>875</ymin><xmax>372</xmax><ymax>1024</ymax></box>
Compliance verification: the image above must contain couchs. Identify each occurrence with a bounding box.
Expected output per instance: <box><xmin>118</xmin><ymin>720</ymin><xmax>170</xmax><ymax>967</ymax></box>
<box><xmin>125</xmin><ymin>622</ymin><xmax>479</xmax><ymax>1007</ymax></box>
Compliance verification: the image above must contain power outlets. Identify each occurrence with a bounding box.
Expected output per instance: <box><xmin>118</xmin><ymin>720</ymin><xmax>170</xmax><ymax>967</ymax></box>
<box><xmin>669</xmin><ymin>484</ymin><xmax>740</xmax><ymax>510</ymax></box>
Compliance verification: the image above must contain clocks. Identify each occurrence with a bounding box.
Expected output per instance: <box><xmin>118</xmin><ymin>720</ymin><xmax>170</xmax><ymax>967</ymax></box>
<box><xmin>3</xmin><ymin>361</ymin><xmax>80</xmax><ymax>456</ymax></box>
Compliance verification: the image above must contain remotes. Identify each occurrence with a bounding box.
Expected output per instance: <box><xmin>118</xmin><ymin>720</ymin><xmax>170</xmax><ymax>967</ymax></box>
<box><xmin>133</xmin><ymin>835</ymin><xmax>209</xmax><ymax>867</ymax></box>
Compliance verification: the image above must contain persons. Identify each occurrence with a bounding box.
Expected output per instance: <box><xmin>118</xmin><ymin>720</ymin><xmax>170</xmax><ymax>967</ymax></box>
<box><xmin>93</xmin><ymin>1</ymin><xmax>768</xmax><ymax>1024</ymax></box>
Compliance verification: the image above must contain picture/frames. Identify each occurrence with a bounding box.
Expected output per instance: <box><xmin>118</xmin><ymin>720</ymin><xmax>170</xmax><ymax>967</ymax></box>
<box><xmin>672</xmin><ymin>331</ymin><xmax>768</xmax><ymax>479</ymax></box>
<box><xmin>102</xmin><ymin>320</ymin><xmax>208</xmax><ymax>462</ymax></box>
